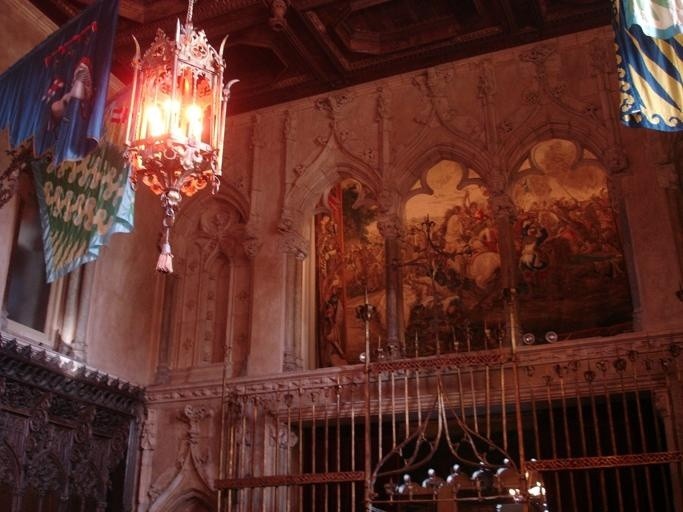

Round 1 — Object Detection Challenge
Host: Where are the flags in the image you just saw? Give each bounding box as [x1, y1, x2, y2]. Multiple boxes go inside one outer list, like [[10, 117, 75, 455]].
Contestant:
[[33, 84, 135, 284], [0, 0, 117, 167], [610, 1, 683, 132]]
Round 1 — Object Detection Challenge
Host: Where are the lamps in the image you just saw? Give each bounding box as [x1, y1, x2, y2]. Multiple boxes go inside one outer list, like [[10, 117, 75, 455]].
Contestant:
[[123, 0, 239, 275], [523, 331, 559, 344]]
